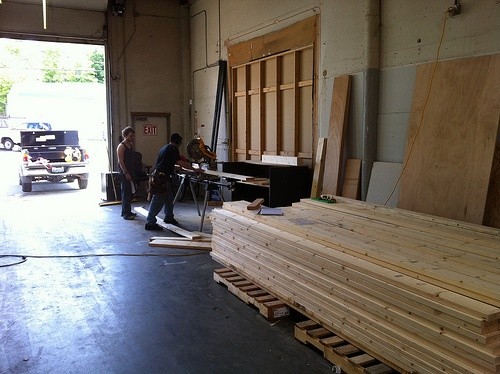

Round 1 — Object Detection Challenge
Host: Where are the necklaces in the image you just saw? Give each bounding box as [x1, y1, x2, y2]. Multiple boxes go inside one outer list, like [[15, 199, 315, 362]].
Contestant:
[[123, 139, 130, 146]]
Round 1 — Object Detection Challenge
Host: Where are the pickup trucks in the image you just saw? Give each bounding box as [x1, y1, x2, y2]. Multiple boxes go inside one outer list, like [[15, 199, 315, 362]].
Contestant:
[[19, 130, 89, 192]]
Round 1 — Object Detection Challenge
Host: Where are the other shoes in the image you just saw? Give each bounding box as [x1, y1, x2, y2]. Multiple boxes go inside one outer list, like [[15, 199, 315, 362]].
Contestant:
[[124, 213, 135, 220], [145, 224, 163, 231], [164, 216, 179, 226]]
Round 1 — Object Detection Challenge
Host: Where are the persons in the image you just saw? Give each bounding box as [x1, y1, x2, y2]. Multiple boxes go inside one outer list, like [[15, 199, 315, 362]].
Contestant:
[[145, 133, 205, 230], [117, 126, 142, 220]]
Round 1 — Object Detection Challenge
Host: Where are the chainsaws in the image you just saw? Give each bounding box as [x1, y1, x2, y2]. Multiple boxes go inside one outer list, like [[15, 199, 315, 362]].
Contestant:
[[187, 137, 218, 168]]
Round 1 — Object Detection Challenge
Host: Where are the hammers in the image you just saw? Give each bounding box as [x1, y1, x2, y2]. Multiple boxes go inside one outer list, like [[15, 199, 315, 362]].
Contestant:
[[146, 172, 153, 201]]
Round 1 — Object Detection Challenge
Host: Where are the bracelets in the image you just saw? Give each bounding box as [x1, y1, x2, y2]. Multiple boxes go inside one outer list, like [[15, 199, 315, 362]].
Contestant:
[[124, 169, 129, 176]]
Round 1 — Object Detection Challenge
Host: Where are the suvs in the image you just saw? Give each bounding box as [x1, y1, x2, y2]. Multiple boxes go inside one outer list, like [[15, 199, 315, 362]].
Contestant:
[[0, 120, 55, 150]]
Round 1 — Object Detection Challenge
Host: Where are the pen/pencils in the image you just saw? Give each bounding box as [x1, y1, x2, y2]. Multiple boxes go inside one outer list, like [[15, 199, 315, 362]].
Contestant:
[[257, 210, 261, 214]]
